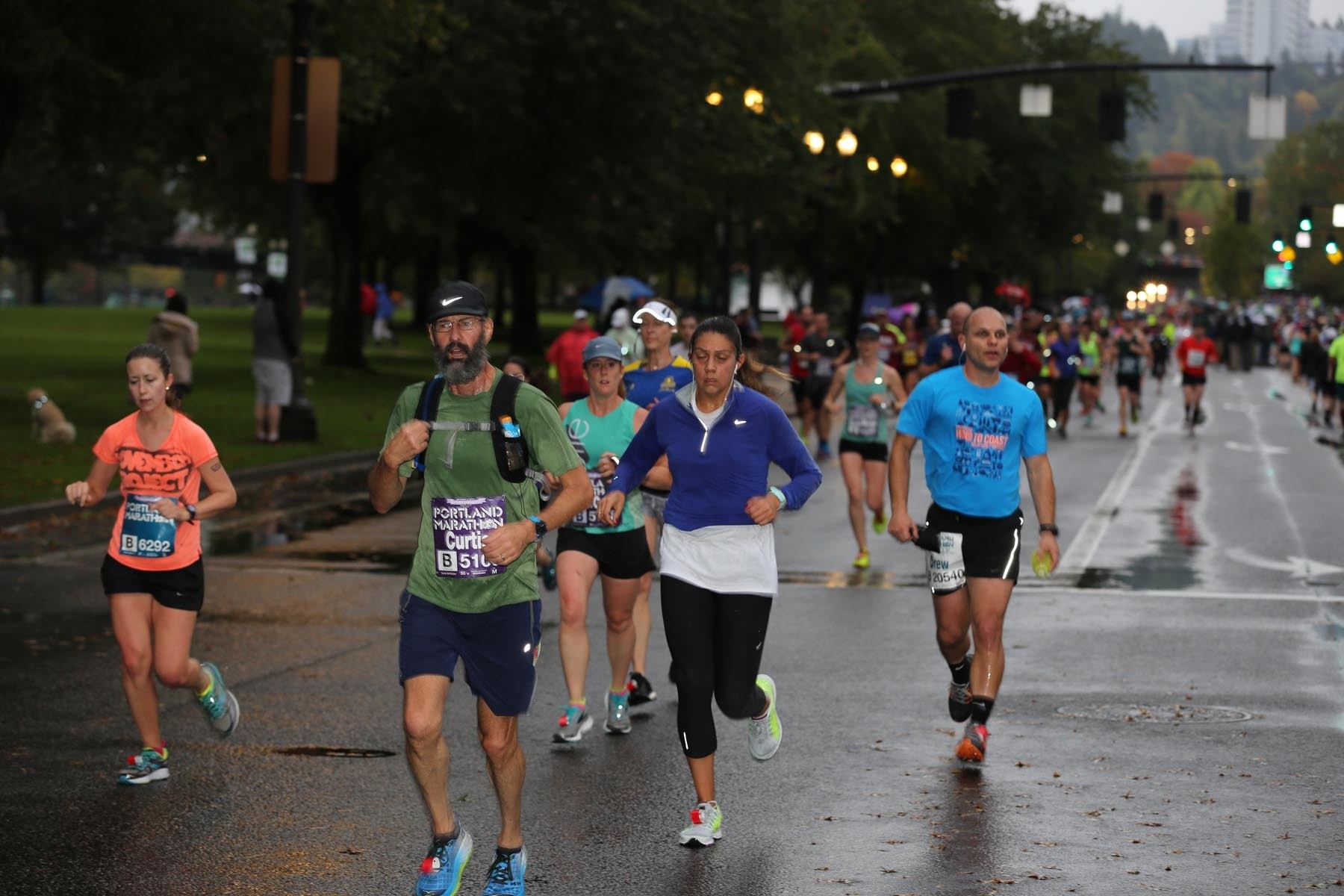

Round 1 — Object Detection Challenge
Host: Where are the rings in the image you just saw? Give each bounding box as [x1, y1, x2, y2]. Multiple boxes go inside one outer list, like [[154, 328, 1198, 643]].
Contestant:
[[500, 554, 505, 561], [598, 512, 601, 514]]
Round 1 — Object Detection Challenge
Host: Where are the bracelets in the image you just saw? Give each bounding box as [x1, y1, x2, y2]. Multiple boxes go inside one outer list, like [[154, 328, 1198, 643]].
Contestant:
[[891, 401, 896, 408], [768, 486, 785, 512]]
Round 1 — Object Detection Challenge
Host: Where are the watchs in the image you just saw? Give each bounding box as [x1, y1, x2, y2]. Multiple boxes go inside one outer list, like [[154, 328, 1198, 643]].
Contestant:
[[183, 504, 196, 525], [529, 515, 548, 539], [1040, 522, 1058, 538]]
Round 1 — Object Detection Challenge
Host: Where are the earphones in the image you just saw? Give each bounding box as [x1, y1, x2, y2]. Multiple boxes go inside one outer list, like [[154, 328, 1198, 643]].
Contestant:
[[962, 343, 966, 351], [734, 363, 738, 374]]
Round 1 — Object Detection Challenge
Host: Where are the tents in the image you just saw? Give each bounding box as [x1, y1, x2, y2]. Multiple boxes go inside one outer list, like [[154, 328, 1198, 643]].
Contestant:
[[573, 278, 653, 331]]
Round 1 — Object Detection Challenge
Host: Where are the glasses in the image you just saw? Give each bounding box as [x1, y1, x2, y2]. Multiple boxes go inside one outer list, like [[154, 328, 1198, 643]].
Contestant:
[[430, 318, 482, 332], [508, 373, 524, 382]]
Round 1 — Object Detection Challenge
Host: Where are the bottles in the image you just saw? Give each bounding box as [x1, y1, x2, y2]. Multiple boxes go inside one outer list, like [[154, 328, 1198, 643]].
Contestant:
[[1031, 550, 1053, 578]]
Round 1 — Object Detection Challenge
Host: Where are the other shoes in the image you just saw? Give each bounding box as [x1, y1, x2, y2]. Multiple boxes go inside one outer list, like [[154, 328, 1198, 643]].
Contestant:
[[853, 548, 869, 568], [255, 437, 281, 445], [873, 503, 887, 534], [814, 448, 830, 465], [541, 551, 557, 591]]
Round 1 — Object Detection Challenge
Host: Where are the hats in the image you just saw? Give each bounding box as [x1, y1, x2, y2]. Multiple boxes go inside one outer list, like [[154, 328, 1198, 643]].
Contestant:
[[573, 309, 587, 319], [856, 322, 880, 340], [581, 336, 622, 367], [632, 301, 678, 327], [610, 307, 629, 327], [424, 279, 488, 324]]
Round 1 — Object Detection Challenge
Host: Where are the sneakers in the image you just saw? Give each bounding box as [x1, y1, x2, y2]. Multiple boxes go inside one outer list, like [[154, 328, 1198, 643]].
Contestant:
[[191, 661, 240, 740], [603, 688, 632, 734], [482, 842, 528, 896], [416, 817, 473, 896], [955, 722, 988, 764], [678, 800, 723, 847], [551, 697, 593, 743], [748, 673, 782, 760], [116, 739, 169, 785], [628, 672, 657, 706], [948, 652, 975, 723]]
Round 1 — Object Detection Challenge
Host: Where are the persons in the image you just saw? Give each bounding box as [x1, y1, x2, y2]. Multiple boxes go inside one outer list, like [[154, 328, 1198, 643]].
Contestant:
[[65, 343, 239, 787], [150, 290, 1344, 895]]
